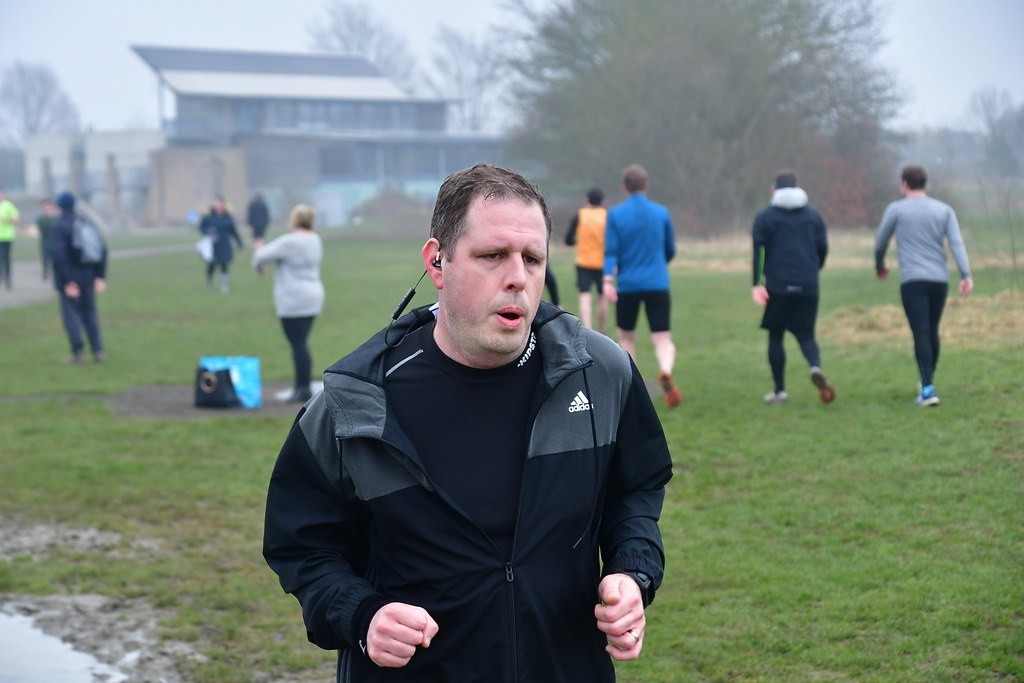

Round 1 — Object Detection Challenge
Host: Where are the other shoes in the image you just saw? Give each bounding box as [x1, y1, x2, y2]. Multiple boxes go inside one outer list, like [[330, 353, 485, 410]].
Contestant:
[[285, 386, 312, 404]]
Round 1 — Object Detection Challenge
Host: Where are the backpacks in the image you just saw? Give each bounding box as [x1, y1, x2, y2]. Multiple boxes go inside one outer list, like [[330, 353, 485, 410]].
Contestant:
[[62, 213, 107, 267]]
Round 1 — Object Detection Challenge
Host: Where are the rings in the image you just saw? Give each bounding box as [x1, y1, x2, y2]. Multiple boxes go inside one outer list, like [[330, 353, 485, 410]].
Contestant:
[[627, 629, 639, 643]]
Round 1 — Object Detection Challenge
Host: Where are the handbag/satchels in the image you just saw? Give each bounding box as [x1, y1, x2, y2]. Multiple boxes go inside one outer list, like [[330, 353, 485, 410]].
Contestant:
[[195, 233, 216, 264], [195, 356, 263, 409]]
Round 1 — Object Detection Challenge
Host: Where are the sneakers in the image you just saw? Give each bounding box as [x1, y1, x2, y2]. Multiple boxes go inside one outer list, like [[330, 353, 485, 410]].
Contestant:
[[916, 380, 940, 407], [658, 371, 681, 407], [809, 366, 836, 404], [763, 390, 788, 404]]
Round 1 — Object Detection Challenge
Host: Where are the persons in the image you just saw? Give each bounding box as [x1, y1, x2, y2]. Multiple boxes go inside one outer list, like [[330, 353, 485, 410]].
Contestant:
[[0, 189, 19, 293], [544, 265, 562, 309], [602, 163, 681, 408], [254, 205, 327, 405], [198, 192, 270, 292], [35, 189, 106, 365], [750, 170, 837, 403], [875, 165, 974, 405], [563, 188, 607, 334], [263, 166, 675, 683]]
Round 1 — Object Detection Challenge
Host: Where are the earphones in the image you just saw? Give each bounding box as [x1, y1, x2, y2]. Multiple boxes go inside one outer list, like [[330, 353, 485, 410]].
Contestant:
[[432, 254, 442, 267]]
